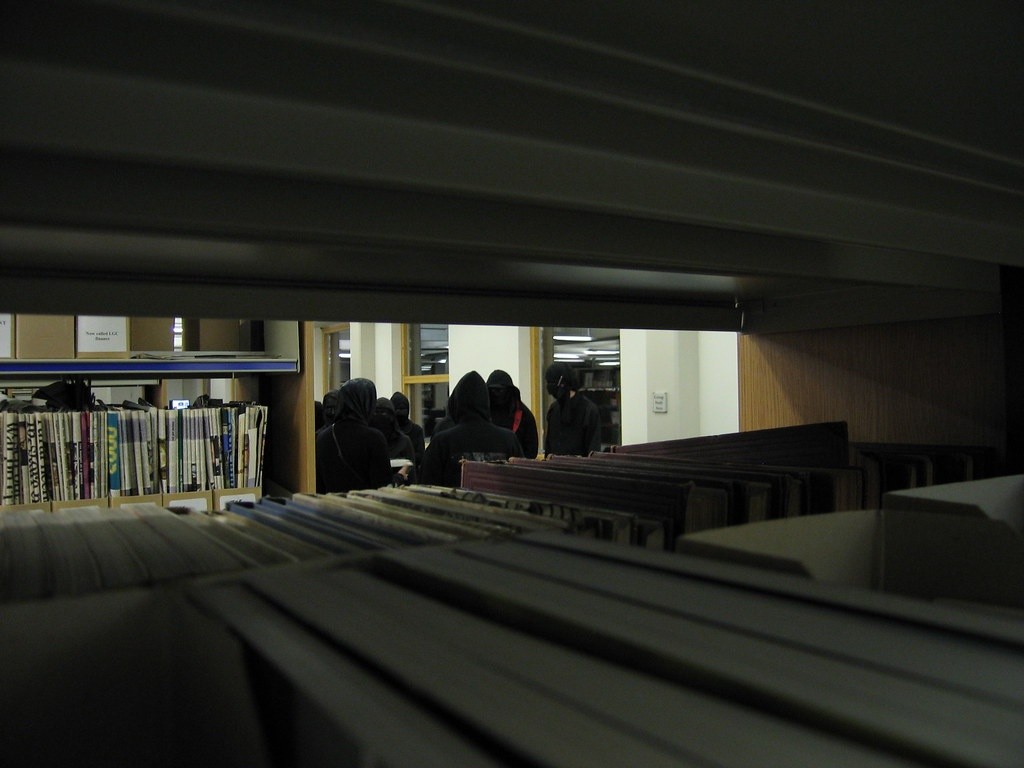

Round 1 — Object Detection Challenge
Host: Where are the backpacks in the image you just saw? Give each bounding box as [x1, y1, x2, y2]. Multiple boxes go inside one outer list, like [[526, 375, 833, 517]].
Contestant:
[[32, 378, 94, 412]]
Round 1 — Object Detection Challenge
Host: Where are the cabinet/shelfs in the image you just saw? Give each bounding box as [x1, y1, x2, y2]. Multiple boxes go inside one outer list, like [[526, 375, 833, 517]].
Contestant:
[[0, 312, 317, 521]]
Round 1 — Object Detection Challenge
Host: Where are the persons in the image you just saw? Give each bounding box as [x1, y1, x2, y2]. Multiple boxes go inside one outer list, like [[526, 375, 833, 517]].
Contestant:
[[315, 368, 540, 496], [539, 361, 603, 458]]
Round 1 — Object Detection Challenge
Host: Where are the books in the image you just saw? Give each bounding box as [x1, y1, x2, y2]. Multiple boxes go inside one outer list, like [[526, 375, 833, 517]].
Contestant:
[[0, 396, 1024, 768]]
